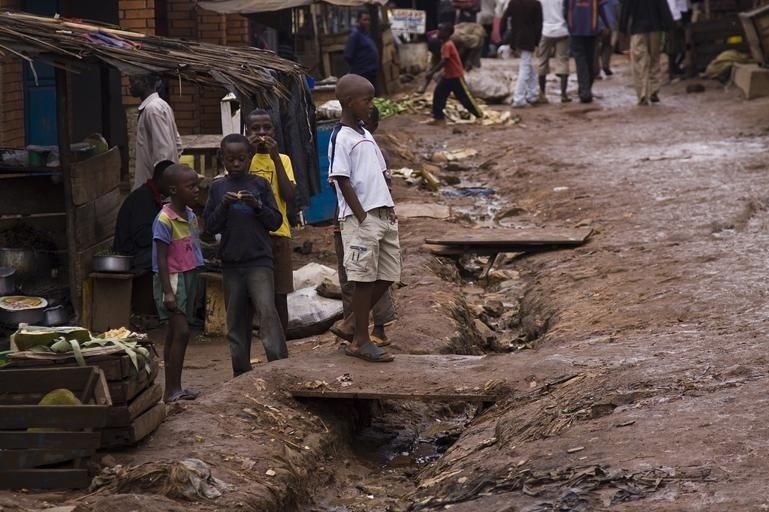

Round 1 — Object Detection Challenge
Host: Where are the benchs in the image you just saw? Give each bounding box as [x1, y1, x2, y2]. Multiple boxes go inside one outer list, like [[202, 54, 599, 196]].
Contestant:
[[86, 265, 149, 335]]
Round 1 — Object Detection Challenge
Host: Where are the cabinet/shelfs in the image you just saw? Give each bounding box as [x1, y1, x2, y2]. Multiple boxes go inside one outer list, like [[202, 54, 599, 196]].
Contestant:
[[737, 4, 769, 67]]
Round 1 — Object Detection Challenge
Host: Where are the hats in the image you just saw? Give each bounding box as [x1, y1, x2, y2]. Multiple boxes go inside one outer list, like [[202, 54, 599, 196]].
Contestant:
[[727, 36, 742, 44]]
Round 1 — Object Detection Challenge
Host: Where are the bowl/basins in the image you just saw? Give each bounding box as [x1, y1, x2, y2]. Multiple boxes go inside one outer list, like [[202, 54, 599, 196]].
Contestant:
[[0, 304, 65, 329], [91, 254, 134, 271]]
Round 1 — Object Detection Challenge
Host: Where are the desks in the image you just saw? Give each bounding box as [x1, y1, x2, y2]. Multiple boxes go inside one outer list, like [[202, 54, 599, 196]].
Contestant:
[[180, 135, 222, 177], [321, 43, 345, 78]]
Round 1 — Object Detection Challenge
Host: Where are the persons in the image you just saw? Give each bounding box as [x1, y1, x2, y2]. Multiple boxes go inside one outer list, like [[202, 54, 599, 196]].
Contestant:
[[111, 160, 175, 269], [360, 1, 498, 69], [620, 1, 692, 105], [151, 164, 205, 403], [332, 104, 399, 348], [328, 74, 402, 363], [425, 21, 483, 124], [128, 73, 184, 193], [203, 134, 288, 377], [498, 1, 619, 103], [245, 109, 297, 341], [343, 11, 379, 94]]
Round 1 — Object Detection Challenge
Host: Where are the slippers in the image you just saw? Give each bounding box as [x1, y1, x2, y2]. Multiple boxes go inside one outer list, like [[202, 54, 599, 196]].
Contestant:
[[641, 96, 659, 106], [163, 389, 197, 401], [369, 335, 391, 346], [603, 67, 613, 75], [329, 326, 354, 343], [513, 95, 592, 108], [344, 341, 395, 362]]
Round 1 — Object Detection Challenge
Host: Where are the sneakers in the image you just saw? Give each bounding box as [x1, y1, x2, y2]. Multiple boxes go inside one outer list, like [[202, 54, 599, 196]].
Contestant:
[[425, 118, 446, 126], [476, 113, 489, 125]]
